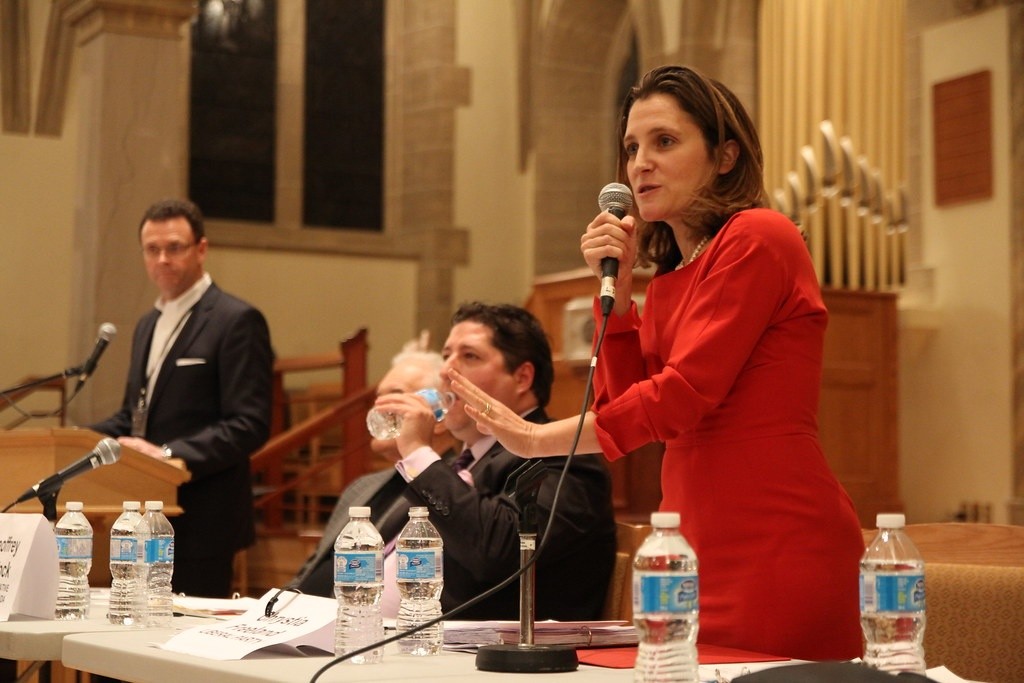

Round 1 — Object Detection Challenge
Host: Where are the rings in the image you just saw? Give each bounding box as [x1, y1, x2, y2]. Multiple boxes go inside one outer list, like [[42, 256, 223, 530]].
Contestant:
[[483, 402, 492, 416]]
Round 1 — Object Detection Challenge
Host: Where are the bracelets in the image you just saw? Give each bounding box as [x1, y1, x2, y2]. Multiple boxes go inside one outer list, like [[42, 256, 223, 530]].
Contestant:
[[161, 442, 174, 458]]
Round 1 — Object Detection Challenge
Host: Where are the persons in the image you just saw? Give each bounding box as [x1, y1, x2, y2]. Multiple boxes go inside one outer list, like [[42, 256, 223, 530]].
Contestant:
[[284, 346, 456, 598], [81, 197, 276, 599], [446, 66, 867, 663], [372, 302, 616, 621]]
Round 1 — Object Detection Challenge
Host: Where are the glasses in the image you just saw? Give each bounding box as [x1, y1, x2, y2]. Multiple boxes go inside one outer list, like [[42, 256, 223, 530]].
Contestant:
[[139, 244, 194, 257]]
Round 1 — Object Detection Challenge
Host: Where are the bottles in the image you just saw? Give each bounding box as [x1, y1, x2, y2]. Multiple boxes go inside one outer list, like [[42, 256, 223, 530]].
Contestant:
[[136, 501, 175, 627], [395, 507, 443, 654], [633, 512, 699, 683], [109, 501, 143, 625], [54, 501, 93, 622], [860, 514, 925, 675], [367, 387, 457, 440], [334, 507, 384, 664]]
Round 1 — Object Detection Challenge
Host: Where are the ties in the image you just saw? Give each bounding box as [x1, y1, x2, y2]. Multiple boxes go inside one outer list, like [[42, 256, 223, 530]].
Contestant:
[[382, 448, 475, 558]]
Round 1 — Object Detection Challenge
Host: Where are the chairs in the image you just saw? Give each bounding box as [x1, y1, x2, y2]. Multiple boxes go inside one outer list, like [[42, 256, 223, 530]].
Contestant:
[[598, 517, 1024, 682]]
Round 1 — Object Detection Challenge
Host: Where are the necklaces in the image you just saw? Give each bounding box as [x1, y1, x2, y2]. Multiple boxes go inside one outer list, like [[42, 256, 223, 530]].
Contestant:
[[675, 232, 710, 272]]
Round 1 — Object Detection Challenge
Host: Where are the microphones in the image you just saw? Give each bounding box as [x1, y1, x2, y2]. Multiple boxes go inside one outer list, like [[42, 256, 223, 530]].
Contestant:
[[598, 183, 633, 317], [16, 438, 123, 503], [75, 321, 115, 391]]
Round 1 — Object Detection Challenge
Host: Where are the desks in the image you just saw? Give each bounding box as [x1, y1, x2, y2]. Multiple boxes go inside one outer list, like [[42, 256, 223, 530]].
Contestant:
[[0, 584, 981, 683]]
